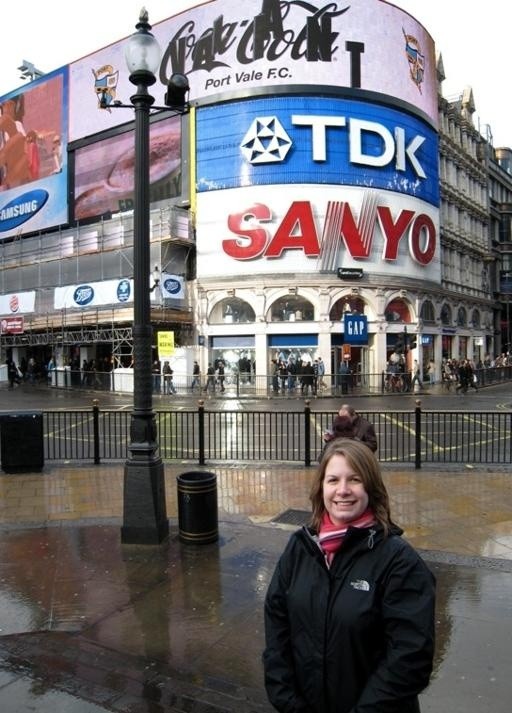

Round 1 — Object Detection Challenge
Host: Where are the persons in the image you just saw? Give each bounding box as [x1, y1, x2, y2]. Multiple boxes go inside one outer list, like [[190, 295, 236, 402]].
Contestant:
[[390, 351, 405, 373], [162, 361, 177, 395], [261, 435, 437, 712], [8, 356, 56, 389], [384, 359, 424, 394], [270, 356, 328, 395], [317, 416, 361, 465], [191, 361, 200, 392], [323, 403, 378, 454], [339, 360, 353, 394], [64, 356, 109, 388], [152, 360, 162, 395], [441, 350, 512, 396], [202, 362, 216, 392], [214, 356, 221, 369], [217, 362, 226, 391], [426, 359, 436, 385]]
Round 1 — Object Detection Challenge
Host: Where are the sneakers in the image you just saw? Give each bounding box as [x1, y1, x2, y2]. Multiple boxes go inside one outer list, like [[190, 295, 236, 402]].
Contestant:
[[167, 387, 225, 395]]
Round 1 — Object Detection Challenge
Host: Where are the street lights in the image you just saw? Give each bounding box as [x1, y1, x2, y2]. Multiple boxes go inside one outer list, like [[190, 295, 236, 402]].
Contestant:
[[100, 6, 191, 545]]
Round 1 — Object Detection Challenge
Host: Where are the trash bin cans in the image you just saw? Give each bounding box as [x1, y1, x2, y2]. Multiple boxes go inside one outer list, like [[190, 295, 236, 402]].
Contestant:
[[177, 471, 218, 545]]
